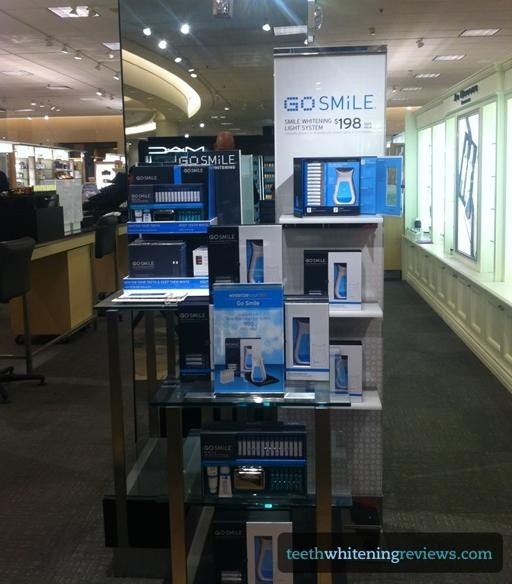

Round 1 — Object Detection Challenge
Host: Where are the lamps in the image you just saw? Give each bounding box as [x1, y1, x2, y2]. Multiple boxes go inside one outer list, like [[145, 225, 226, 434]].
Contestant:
[[27, 1, 122, 123], [144, 21, 271, 139]]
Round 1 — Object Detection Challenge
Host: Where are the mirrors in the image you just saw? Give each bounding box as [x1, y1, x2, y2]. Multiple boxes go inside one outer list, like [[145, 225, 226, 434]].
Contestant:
[[115, 0, 309, 166]]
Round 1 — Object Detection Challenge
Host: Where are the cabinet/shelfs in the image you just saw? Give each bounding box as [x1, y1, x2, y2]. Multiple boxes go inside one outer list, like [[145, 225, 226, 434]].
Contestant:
[[92, 45, 387, 583], [8, 144, 87, 187]]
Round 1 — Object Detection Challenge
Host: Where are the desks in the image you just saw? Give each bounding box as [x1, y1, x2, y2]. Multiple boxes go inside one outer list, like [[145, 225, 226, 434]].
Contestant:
[[8, 225, 127, 345]]
